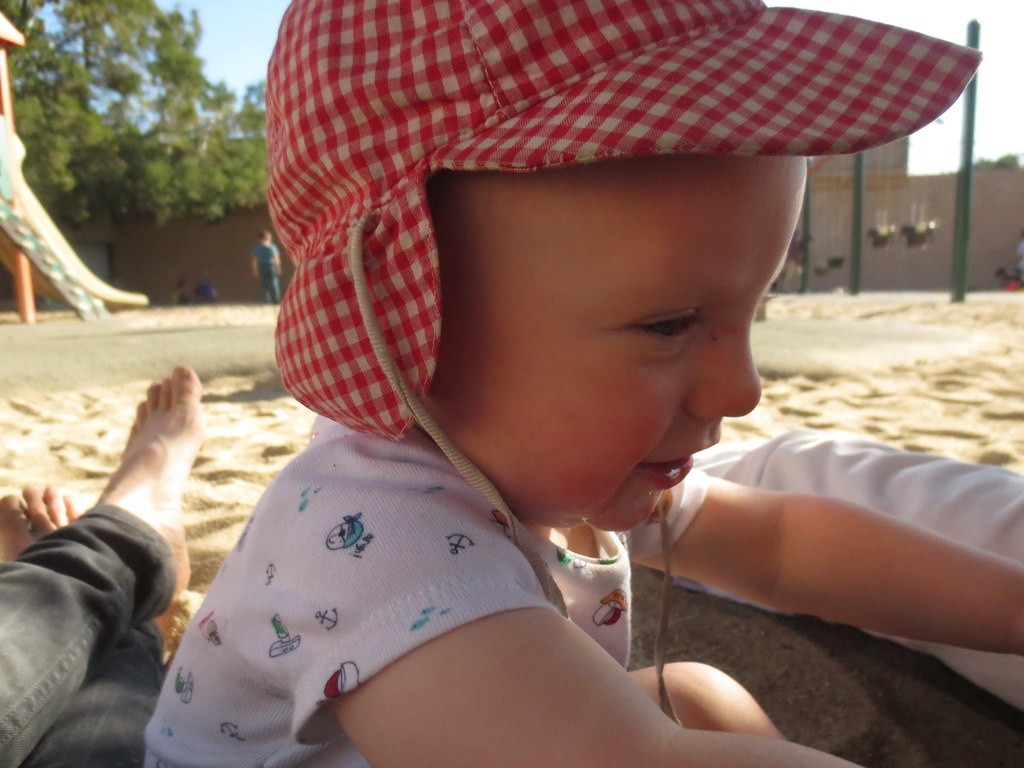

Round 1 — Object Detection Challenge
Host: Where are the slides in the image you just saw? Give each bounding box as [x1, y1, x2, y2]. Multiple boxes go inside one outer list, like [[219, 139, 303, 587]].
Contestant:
[[1, 115, 151, 311]]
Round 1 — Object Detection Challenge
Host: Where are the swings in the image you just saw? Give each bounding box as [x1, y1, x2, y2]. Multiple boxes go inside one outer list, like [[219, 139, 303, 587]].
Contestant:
[[813, 125, 935, 277]]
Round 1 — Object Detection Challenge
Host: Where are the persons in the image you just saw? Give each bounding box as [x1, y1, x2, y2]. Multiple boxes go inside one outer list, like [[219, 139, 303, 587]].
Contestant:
[[0, 365, 203, 768], [250, 231, 282, 304], [143, 1, 1024, 768]]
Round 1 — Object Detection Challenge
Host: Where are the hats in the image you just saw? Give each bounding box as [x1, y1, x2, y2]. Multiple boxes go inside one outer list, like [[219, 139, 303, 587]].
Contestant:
[[259, 0, 981, 442]]
